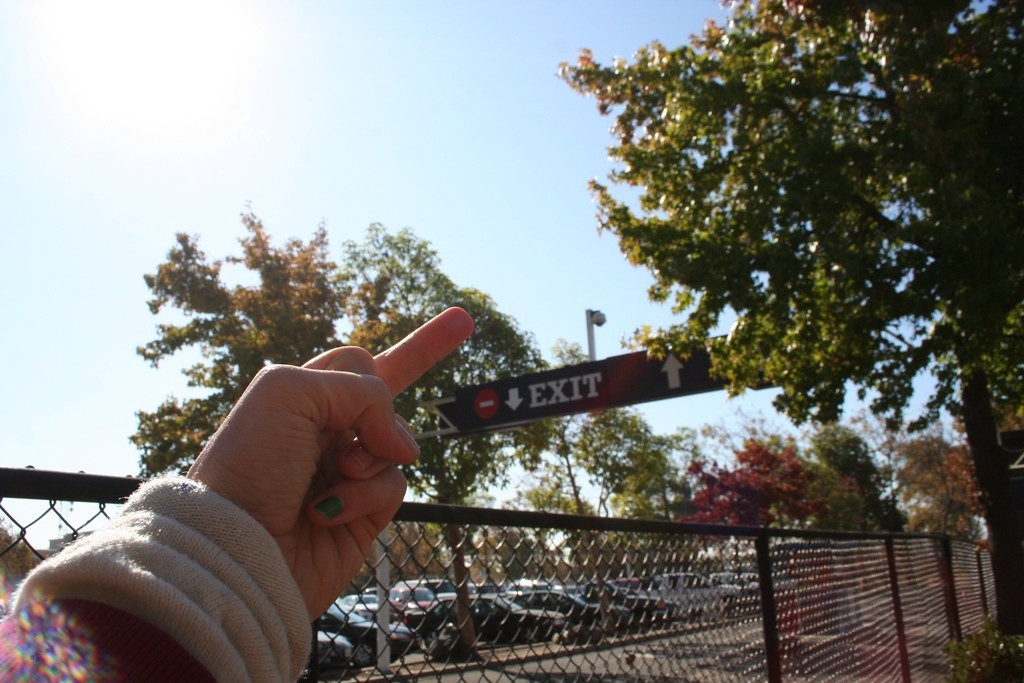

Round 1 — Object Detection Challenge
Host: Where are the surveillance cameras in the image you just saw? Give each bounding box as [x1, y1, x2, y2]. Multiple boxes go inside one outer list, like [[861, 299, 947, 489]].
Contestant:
[[593, 312, 606, 326]]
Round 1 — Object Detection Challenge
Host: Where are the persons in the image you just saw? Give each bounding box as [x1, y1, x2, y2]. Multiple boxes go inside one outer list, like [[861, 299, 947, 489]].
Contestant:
[[0, 307, 475, 683]]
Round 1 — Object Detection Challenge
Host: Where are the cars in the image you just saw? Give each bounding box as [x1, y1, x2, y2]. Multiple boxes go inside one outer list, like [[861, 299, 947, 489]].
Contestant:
[[309, 559, 798, 679]]
